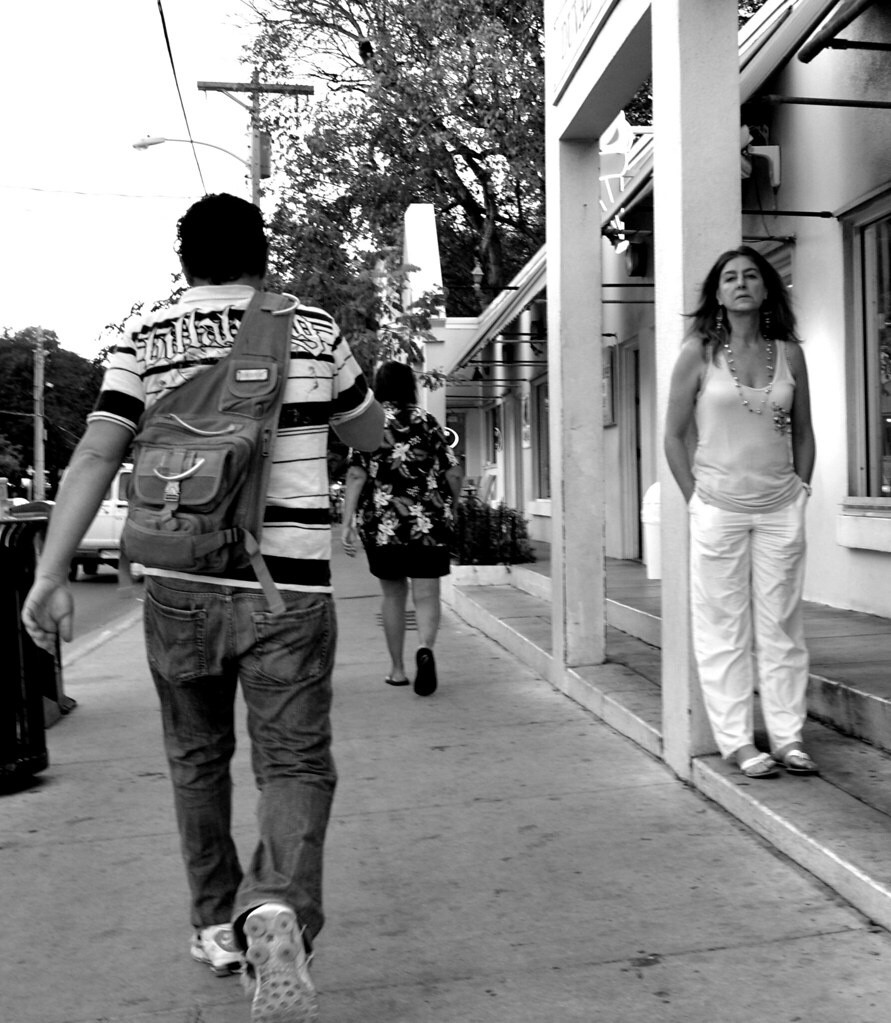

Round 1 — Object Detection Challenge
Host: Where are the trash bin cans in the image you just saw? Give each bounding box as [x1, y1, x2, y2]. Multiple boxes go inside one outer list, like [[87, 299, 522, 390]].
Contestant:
[[0, 516, 51, 786]]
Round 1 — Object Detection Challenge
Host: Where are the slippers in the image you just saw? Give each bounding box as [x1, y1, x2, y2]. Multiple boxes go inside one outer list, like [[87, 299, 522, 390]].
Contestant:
[[384, 672, 410, 686], [414, 648, 438, 697], [783, 747, 818, 776], [740, 753, 781, 779]]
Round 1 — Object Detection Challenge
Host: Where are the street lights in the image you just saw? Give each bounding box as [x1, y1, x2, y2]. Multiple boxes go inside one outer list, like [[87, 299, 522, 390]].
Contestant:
[[133, 137, 261, 220]]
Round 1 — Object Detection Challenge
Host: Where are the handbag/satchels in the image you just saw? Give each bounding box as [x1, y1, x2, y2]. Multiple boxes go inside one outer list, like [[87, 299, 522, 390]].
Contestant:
[[116, 289, 300, 578]]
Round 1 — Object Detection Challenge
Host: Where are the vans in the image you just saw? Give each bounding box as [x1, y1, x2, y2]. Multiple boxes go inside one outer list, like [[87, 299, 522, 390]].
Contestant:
[[68, 462, 134, 582]]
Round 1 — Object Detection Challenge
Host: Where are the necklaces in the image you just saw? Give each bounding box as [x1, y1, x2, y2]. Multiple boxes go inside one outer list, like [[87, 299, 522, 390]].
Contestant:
[[724, 334, 774, 414]]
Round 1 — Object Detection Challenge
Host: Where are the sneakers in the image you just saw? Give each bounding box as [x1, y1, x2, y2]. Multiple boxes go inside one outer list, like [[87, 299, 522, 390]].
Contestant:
[[190, 922, 248, 976], [244, 902, 316, 1023]]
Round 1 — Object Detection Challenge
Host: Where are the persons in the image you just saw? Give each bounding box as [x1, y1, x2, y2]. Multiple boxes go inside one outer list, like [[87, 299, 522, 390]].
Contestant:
[[20, 194, 385, 1023], [342, 362, 464, 697], [664, 249, 819, 776]]
